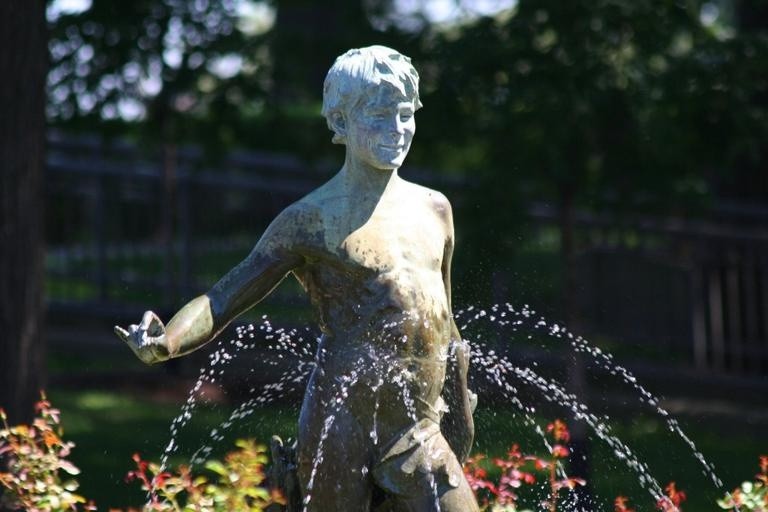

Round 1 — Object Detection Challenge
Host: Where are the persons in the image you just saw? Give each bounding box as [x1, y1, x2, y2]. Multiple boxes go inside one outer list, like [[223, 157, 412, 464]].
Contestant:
[[112, 44, 483, 512]]
